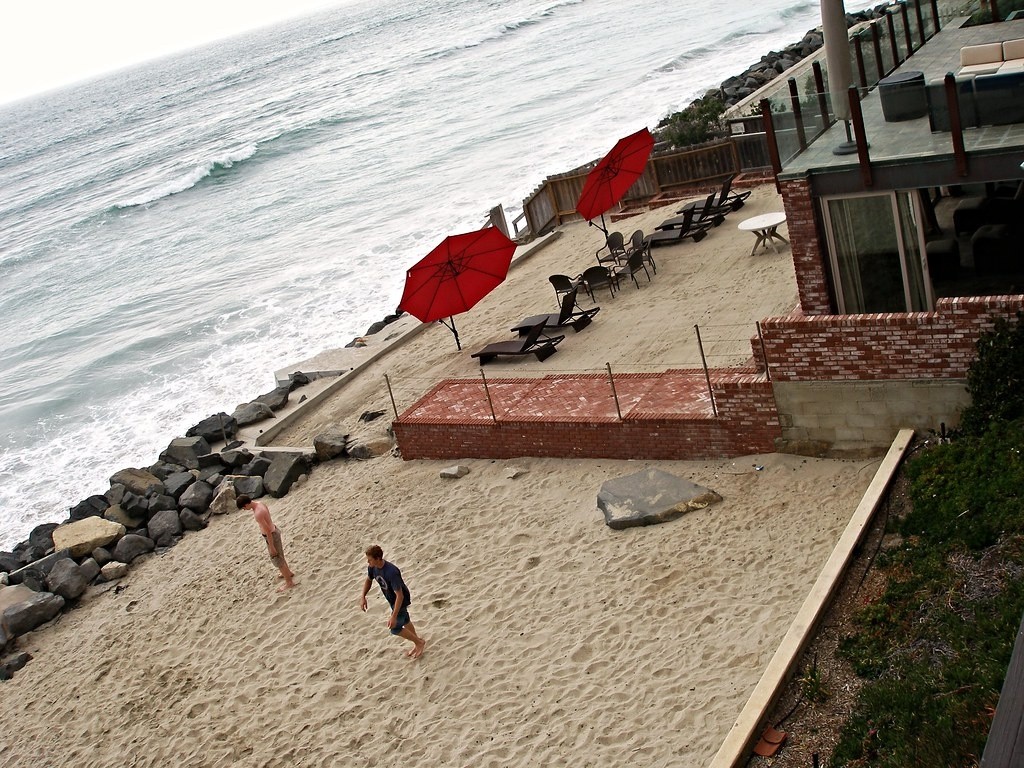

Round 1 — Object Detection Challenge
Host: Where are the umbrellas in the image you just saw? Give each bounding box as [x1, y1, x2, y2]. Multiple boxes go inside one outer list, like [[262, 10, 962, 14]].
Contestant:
[[396, 224, 518, 349], [576, 127, 658, 242]]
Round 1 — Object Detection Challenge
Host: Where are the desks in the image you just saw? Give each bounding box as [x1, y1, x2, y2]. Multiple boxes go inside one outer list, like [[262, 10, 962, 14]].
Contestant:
[[878, 71, 927, 122], [738, 212, 787, 256]]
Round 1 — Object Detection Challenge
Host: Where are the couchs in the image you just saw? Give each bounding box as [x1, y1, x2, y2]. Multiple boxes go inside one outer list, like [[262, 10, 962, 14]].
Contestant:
[[925, 38, 1024, 134], [989, 181, 1024, 208]]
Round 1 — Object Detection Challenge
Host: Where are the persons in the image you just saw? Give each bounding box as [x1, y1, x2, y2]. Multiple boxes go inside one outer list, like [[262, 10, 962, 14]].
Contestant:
[[234, 494, 294, 587], [359, 545, 427, 659]]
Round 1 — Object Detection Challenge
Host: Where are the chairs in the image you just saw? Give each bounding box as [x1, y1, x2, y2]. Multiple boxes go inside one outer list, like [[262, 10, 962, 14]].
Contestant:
[[583, 266, 616, 303], [510, 287, 600, 338], [643, 203, 707, 242], [676, 174, 751, 211], [596, 232, 626, 266], [613, 249, 650, 290], [654, 191, 725, 228], [549, 273, 590, 308], [629, 235, 656, 281], [615, 230, 643, 266], [471, 316, 565, 366]]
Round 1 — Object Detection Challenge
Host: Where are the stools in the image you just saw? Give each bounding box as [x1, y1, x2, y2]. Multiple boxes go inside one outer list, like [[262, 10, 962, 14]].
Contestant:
[[970, 224, 1008, 274], [953, 196, 987, 239], [926, 239, 960, 268]]
